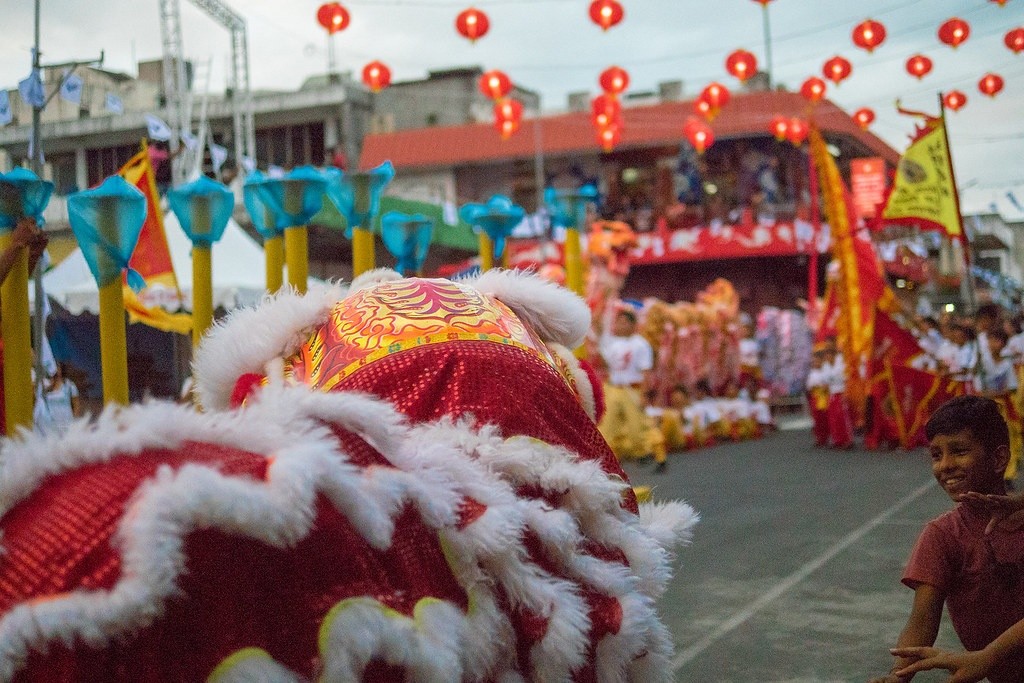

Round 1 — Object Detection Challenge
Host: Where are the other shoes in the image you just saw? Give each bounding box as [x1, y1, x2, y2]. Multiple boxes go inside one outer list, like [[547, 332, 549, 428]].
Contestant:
[[658, 463, 667, 473]]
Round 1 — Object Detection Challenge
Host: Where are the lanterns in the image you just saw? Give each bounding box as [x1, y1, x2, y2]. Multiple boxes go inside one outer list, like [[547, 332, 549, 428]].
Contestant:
[[317, 0, 1024, 152]]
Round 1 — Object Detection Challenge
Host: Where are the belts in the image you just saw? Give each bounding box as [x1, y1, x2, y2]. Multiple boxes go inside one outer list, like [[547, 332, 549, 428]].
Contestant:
[[616, 384, 642, 388]]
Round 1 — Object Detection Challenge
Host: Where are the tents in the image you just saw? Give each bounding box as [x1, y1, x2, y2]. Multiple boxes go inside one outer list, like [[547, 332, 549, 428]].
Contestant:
[[35, 185, 326, 405]]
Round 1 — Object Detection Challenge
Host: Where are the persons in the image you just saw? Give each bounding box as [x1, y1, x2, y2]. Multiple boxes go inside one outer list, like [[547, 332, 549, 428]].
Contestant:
[[580, 255, 1024, 493], [869, 393, 1024, 683], [888, 615, 1024, 683]]
